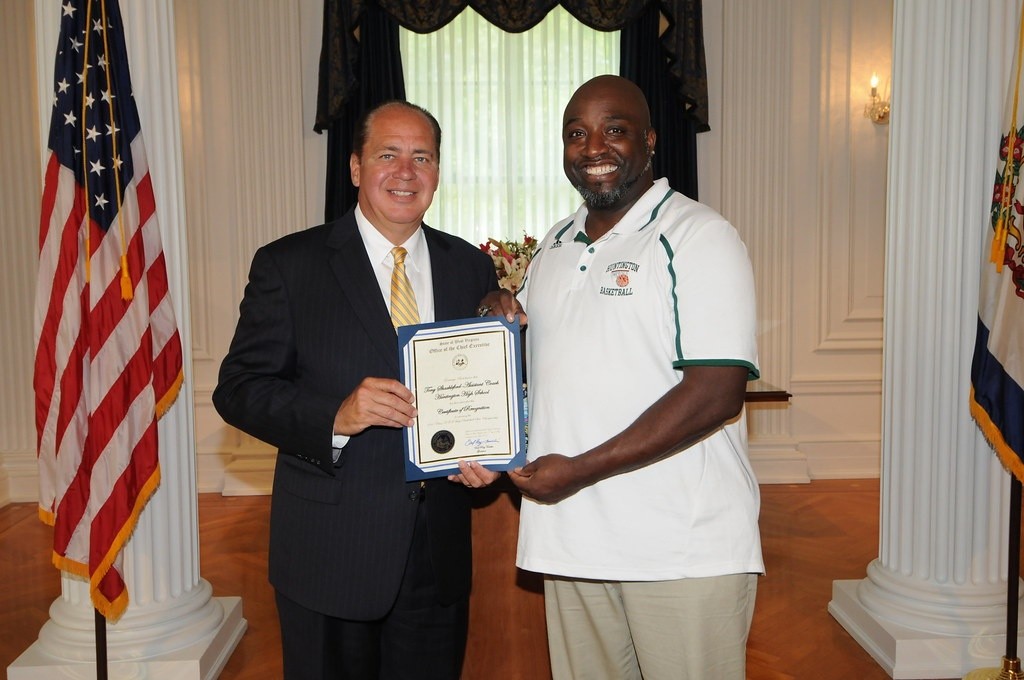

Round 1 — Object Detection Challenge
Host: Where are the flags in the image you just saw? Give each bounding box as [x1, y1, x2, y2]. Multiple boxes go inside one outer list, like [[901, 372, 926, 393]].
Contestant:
[[33, 1, 184, 625], [968, 0, 1024, 484]]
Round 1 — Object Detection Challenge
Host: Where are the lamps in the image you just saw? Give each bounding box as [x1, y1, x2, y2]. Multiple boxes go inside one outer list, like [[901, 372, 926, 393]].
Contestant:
[[864, 70, 891, 125]]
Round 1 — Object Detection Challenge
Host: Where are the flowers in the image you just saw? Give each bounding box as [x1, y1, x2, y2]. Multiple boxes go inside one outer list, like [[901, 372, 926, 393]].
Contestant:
[[479, 234, 538, 295]]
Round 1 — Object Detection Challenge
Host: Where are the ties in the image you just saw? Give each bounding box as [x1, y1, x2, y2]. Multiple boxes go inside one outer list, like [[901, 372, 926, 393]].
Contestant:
[[390, 246, 420, 328]]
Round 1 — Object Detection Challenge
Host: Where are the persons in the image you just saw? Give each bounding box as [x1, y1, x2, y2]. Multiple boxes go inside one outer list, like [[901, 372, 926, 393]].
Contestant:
[[477, 75, 767, 680], [212, 100, 501, 680]]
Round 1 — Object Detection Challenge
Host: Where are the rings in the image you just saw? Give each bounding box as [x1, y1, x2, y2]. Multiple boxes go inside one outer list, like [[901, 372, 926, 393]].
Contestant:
[[477, 303, 491, 316]]
